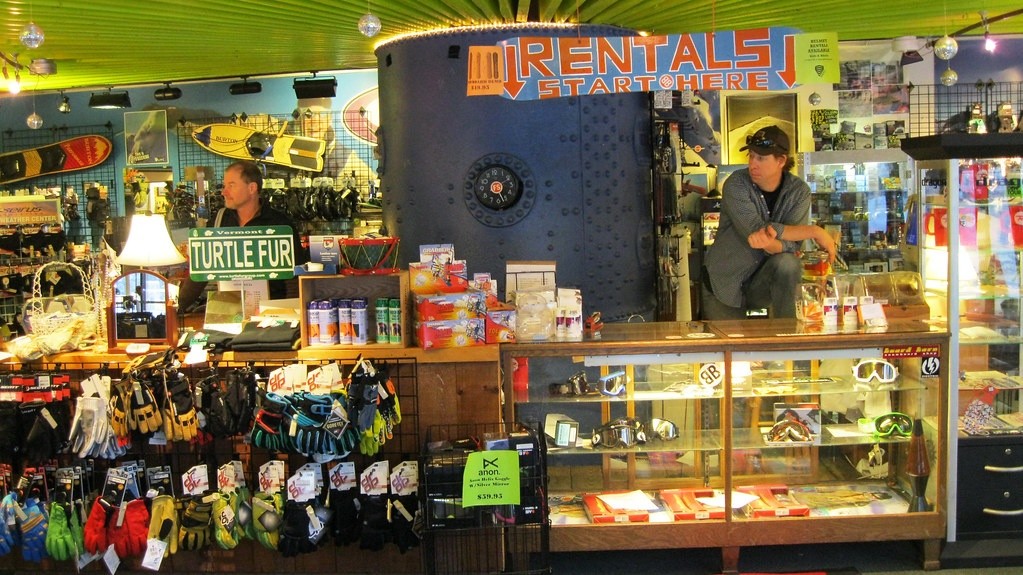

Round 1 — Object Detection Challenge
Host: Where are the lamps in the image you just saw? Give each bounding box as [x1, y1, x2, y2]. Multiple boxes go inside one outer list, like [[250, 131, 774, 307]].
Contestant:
[[292, 71, 338, 99], [359, 0, 381, 38], [934, 1, 959, 59], [89, 88, 132, 110], [984, 17, 994, 50], [154, 82, 182, 100], [230, 76, 262, 95], [116, 213, 188, 272], [809, 91, 820, 105], [20, 1, 45, 48], [940, 59, 958, 87], [58, 91, 71, 114], [2, 65, 20, 94], [27, 93, 44, 130]]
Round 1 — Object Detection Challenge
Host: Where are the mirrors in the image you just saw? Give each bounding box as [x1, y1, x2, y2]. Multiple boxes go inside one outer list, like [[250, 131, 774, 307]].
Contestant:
[[107, 269, 168, 353]]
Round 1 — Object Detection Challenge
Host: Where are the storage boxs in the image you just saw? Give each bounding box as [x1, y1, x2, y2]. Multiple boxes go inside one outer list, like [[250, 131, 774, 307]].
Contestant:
[[736, 486, 808, 516], [660, 489, 725, 521], [408, 245, 583, 349], [582, 491, 650, 524], [299, 271, 411, 350], [545, 414, 580, 447], [774, 402, 822, 434]]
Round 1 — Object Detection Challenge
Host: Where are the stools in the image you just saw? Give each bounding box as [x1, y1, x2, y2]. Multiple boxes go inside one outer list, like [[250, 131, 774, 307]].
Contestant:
[[741, 358, 819, 474]]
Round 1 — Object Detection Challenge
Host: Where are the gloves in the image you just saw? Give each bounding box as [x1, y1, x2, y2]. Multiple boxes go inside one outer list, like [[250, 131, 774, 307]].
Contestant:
[[0, 366, 420, 560]]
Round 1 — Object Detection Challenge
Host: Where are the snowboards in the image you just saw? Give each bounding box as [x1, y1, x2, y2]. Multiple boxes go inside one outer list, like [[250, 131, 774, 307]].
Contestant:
[[191, 124, 327, 172], [0, 135, 112, 185]]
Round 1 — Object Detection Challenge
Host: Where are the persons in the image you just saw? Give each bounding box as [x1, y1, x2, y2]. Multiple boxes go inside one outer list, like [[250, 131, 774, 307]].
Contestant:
[[205, 161, 305, 300], [701, 126, 835, 320]]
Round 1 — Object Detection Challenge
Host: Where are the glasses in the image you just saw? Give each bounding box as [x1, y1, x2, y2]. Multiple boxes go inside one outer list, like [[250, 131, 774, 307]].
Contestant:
[[851, 359, 899, 383], [858, 413, 915, 436], [549, 371, 591, 395], [592, 416, 679, 448], [598, 371, 627, 396], [746, 135, 788, 150], [771, 421, 814, 443]]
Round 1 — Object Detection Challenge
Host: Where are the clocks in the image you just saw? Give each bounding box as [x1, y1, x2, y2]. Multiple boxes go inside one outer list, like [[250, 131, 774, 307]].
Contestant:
[[475, 168, 516, 207]]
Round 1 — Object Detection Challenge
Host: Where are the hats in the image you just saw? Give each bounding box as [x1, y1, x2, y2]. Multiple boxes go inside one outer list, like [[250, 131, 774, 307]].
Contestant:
[[739, 125, 789, 152]]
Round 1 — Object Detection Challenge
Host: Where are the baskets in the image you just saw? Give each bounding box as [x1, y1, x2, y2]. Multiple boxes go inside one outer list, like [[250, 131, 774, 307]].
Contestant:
[[29, 261, 97, 336]]
